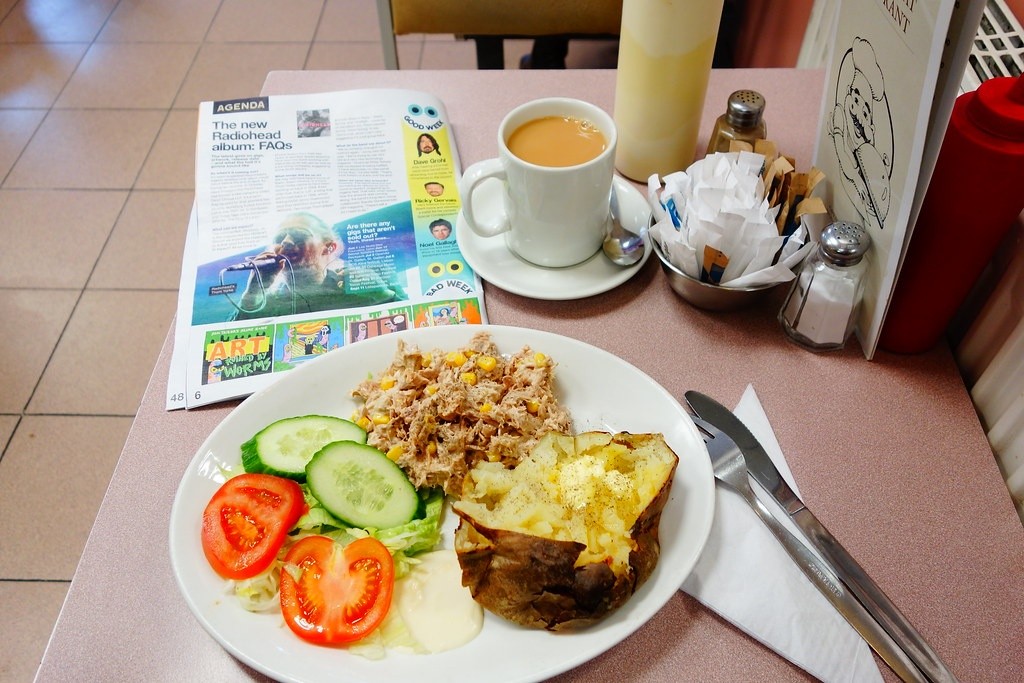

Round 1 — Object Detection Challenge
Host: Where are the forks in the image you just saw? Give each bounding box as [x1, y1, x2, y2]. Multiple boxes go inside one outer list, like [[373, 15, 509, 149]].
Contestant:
[[686, 413, 929, 683]]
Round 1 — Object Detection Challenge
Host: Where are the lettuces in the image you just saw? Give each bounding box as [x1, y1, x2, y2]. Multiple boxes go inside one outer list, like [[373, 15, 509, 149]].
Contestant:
[[215, 464, 443, 655]]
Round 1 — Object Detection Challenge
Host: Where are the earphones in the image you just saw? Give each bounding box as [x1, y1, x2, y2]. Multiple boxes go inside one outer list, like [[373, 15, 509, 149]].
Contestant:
[[326, 245, 333, 252]]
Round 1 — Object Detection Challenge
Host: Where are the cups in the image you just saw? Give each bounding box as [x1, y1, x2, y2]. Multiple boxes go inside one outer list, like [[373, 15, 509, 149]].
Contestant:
[[459, 97, 618, 268]]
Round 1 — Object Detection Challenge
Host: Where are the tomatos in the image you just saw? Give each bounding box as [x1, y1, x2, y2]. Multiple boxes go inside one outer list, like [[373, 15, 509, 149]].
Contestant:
[[200, 473, 307, 580], [278, 536, 394, 645]]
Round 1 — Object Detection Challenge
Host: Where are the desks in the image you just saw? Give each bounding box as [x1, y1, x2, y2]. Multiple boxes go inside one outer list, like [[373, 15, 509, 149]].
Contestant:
[[33, 67, 1023, 683]]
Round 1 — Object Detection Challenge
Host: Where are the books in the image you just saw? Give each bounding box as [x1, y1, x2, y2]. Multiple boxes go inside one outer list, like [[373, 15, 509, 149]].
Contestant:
[[165, 89, 491, 411]]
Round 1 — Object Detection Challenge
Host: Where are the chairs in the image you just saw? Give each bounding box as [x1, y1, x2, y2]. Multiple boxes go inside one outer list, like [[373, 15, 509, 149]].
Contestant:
[[376, 0, 624, 69]]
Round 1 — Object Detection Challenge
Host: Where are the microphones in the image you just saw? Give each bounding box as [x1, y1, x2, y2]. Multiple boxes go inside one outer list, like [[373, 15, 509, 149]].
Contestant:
[[226, 255, 285, 271]]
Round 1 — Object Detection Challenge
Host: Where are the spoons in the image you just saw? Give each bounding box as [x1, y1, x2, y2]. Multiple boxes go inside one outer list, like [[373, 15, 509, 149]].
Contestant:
[[602, 183, 645, 266]]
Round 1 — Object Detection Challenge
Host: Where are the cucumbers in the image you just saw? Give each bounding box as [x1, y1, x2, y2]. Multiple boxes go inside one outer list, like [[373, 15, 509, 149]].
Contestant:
[[305, 440, 427, 530], [240, 415, 369, 483]]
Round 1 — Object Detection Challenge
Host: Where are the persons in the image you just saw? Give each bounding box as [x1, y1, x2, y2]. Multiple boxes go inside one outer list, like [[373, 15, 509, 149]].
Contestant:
[[428, 218, 451, 241], [417, 133, 441, 158], [225, 212, 404, 321], [424, 181, 444, 197]]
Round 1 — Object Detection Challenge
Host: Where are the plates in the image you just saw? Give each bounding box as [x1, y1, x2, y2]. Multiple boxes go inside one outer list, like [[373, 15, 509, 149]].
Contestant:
[[454, 174, 654, 301], [167, 325, 715, 683]]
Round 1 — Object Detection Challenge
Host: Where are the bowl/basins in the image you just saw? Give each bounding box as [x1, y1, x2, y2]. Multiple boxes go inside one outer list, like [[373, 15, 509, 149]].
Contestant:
[[648, 207, 804, 310]]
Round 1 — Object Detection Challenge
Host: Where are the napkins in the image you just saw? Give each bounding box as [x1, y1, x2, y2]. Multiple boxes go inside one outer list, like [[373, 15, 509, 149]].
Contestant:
[[678, 384, 884, 683]]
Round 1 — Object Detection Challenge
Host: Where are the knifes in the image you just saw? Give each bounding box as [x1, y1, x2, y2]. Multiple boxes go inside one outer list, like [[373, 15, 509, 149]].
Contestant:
[[686, 389, 963, 683]]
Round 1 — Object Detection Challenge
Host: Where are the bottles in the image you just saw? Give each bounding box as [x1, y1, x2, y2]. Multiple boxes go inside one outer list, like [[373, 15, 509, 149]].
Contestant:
[[777, 221, 872, 351], [703, 89, 767, 158], [878, 68, 1024, 357]]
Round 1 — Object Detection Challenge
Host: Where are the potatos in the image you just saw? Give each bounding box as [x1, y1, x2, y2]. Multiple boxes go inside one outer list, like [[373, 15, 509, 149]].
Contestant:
[[450, 430, 679, 633]]
